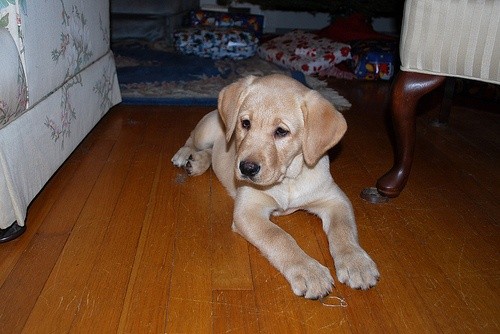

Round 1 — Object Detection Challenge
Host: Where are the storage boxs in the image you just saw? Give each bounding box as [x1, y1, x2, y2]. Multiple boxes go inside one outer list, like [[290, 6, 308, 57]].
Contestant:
[[258, 29, 352, 77], [176, 25, 257, 58]]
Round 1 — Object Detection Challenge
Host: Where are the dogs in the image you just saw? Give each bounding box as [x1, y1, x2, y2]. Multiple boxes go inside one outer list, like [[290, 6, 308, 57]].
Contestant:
[[171, 74, 381, 300]]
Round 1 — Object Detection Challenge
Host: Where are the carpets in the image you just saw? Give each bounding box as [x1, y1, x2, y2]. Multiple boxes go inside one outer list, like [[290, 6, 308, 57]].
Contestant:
[[111, 39, 358, 111]]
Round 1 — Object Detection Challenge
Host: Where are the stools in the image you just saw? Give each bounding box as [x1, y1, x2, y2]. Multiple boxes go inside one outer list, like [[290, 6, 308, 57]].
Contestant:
[[375, 0, 500, 198]]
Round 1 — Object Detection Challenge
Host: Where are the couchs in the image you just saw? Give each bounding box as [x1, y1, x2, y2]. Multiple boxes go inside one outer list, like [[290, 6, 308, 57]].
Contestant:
[[110, 0, 200, 39], [0, 0, 123, 243]]
